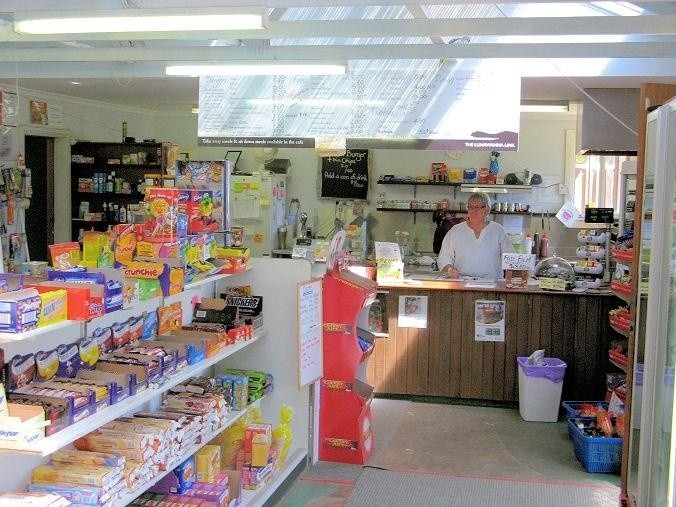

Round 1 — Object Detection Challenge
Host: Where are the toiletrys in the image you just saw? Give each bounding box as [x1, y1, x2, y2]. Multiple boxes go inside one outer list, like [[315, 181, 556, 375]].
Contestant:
[[91, 171, 116, 193], [102, 202, 126, 223]]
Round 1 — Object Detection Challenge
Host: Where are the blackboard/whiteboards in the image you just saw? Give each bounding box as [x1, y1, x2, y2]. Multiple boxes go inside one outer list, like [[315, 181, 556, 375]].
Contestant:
[[296, 275, 324, 391], [317, 148, 372, 203]]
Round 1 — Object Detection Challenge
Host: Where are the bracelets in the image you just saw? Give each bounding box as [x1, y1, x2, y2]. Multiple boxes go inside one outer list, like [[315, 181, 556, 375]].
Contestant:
[[447, 266, 454, 273]]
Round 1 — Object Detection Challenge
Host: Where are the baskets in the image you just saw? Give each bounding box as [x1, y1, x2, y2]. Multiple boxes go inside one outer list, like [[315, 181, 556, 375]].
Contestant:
[[561, 400, 628, 473]]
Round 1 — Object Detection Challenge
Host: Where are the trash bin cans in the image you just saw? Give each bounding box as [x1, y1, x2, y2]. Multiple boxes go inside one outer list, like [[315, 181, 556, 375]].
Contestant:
[[517, 357, 565, 422]]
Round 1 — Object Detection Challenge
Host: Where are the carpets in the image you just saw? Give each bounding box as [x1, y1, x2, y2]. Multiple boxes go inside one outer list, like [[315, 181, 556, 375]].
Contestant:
[[345, 466, 621, 507]]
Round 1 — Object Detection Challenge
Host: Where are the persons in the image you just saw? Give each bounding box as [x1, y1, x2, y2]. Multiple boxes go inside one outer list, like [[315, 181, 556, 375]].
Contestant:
[[436, 191, 516, 279]]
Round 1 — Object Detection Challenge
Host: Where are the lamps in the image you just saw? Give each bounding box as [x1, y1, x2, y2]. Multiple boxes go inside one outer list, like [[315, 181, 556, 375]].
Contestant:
[[521, 100, 569, 112], [13, 6, 270, 35], [164, 59, 349, 77]]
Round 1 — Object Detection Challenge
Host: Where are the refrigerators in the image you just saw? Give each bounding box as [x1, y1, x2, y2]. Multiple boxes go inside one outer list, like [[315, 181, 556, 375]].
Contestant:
[[228, 171, 287, 260]]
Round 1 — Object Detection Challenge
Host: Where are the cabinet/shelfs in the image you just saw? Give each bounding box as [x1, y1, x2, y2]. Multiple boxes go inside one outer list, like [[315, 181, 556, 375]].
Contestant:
[[318, 270, 378, 465], [70, 142, 166, 250], [0, 258, 311, 507], [604, 250, 633, 408], [377, 179, 534, 224]]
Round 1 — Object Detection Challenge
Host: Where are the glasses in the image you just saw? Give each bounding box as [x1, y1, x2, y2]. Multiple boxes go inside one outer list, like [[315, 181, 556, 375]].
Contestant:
[[466, 204, 486, 209]]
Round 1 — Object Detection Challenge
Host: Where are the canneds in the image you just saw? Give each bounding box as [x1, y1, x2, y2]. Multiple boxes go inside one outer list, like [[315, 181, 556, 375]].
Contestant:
[[523, 169, 531, 185], [491, 201, 519, 211], [454, 202, 467, 211]]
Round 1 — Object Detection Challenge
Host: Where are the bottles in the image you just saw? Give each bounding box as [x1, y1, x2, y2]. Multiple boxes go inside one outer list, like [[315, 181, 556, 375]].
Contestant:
[[525, 228, 550, 257], [92, 171, 116, 193], [101, 201, 127, 223]]
